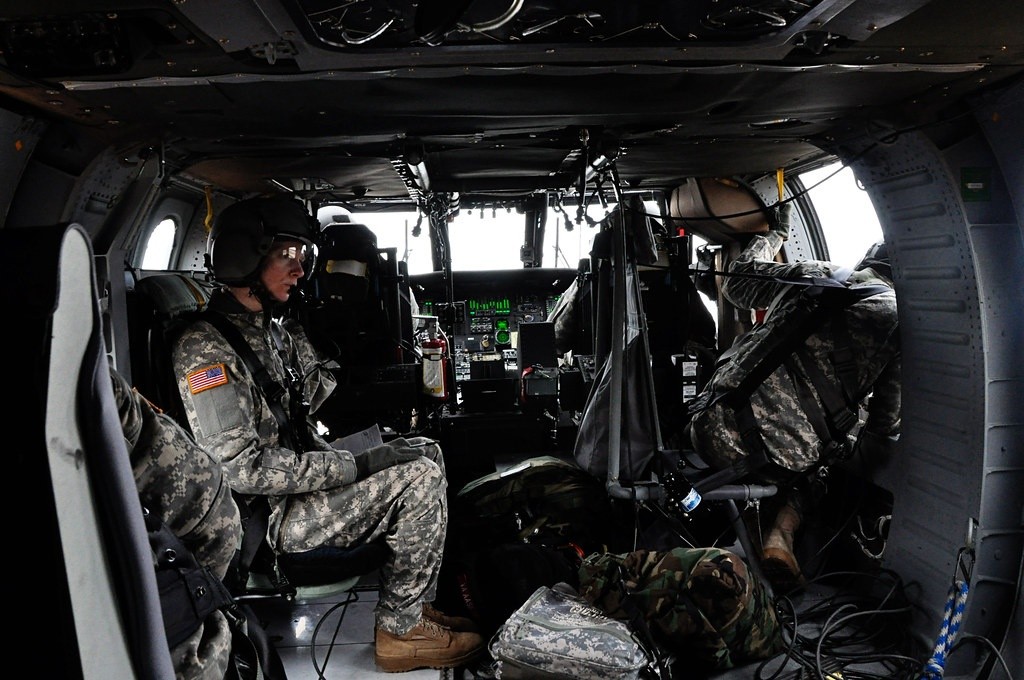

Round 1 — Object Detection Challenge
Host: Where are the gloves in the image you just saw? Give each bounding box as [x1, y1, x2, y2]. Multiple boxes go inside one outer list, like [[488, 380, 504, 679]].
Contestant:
[[352, 437, 426, 483], [763, 201, 790, 233]]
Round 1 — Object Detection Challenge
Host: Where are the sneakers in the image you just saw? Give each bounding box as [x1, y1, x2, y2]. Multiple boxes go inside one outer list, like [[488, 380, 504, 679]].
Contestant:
[[420, 603, 471, 631], [374, 615, 482, 672]]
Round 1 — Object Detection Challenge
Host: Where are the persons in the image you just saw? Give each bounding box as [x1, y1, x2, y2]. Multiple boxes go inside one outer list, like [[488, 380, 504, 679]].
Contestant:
[[158, 191, 480, 672], [688, 240, 902, 583], [108, 364, 243, 680]]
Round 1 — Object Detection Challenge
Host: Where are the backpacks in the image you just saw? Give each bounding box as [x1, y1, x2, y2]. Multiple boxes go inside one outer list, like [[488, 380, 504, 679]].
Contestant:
[[489, 581, 675, 680]]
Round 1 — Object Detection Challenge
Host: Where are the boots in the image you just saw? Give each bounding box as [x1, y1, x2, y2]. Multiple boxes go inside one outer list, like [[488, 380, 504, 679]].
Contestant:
[[761, 507, 809, 591]]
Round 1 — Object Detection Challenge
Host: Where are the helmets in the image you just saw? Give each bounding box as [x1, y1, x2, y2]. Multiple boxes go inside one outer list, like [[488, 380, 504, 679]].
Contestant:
[[212, 197, 318, 287], [855, 239, 890, 273]]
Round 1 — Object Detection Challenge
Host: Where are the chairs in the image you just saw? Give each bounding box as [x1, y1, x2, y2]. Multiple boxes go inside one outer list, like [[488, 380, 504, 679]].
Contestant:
[[574, 220, 689, 409], [307, 223, 403, 425], [0, 220, 270, 680], [133, 273, 384, 600]]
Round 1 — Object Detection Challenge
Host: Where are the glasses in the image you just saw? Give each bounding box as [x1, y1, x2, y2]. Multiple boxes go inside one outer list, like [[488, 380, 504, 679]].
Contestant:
[[270, 248, 307, 269]]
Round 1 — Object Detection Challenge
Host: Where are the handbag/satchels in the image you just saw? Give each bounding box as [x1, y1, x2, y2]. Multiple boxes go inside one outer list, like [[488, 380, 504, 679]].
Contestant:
[[578, 547, 785, 677]]
